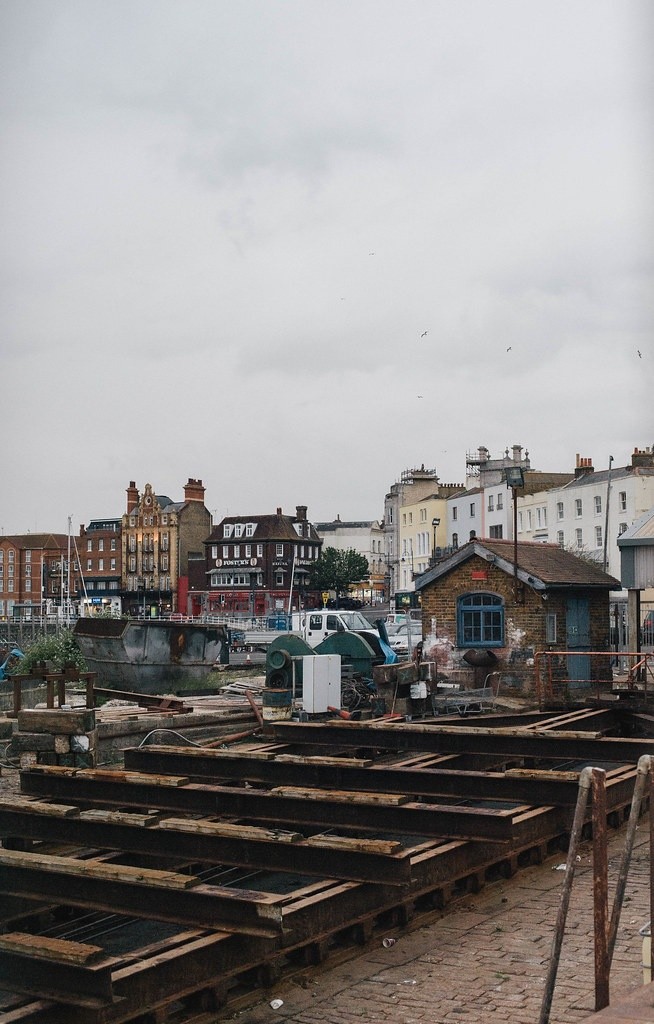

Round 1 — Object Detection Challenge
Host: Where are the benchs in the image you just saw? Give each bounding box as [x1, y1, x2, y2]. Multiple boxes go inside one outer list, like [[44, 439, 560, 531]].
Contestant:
[[311, 623, 335, 630]]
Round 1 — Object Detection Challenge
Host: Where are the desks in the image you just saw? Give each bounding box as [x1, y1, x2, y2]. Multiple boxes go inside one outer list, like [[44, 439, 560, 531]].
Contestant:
[[10, 672, 98, 718]]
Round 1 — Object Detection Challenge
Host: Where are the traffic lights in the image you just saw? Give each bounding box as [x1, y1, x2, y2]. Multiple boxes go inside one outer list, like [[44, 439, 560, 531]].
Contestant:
[[198, 597, 201, 606], [218, 594, 221, 604], [250, 593, 254, 604], [201, 596, 205, 605], [221, 594, 225, 603]]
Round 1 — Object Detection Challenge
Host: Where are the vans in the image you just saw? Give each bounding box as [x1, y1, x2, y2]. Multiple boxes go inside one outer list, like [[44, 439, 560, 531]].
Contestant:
[[382, 608, 423, 637]]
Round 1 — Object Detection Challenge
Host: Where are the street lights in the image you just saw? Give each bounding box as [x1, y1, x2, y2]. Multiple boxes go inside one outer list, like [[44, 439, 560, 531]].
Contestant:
[[502, 466, 525, 601], [602, 454, 614, 573], [149, 578, 155, 619]]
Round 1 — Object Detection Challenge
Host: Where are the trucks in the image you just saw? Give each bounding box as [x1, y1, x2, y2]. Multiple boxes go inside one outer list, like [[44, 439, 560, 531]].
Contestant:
[[243, 611, 379, 653]]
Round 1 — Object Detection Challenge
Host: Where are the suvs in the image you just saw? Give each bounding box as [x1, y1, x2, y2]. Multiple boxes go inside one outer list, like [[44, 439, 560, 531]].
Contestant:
[[332, 597, 363, 610], [643, 610, 654, 645]]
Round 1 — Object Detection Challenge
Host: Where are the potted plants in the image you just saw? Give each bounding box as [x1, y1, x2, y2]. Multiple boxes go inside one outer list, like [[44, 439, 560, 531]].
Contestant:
[[10, 633, 49, 676], [50, 645, 88, 674]]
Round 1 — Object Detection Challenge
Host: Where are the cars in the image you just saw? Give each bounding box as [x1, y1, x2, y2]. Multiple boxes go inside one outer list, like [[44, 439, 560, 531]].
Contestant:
[[387, 622, 422, 656]]
